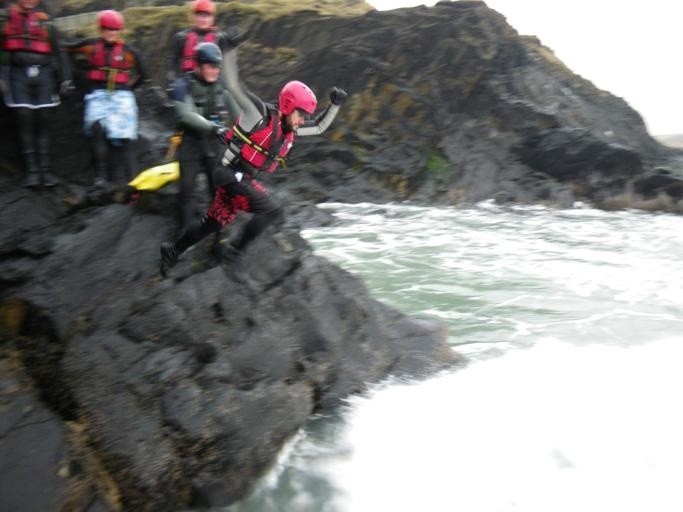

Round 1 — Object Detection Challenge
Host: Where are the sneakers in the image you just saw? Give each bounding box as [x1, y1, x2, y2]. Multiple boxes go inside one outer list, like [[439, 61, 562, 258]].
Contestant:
[[22, 174, 58, 187], [160, 241, 178, 278]]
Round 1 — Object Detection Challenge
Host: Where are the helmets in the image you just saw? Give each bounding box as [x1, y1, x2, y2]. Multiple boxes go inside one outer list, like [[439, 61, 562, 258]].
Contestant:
[[97, 9, 124, 29], [192, 0, 215, 14], [279, 80, 318, 115], [193, 42, 223, 67]]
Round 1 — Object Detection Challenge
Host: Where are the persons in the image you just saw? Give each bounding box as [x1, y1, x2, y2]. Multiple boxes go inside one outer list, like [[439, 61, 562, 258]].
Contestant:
[[0, 0, 74, 188], [78, 8, 147, 198], [159, 1, 348, 279]]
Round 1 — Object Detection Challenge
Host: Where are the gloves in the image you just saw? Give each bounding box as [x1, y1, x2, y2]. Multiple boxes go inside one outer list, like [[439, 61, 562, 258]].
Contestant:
[[330, 86, 347, 105], [212, 125, 230, 145], [218, 31, 239, 51]]
